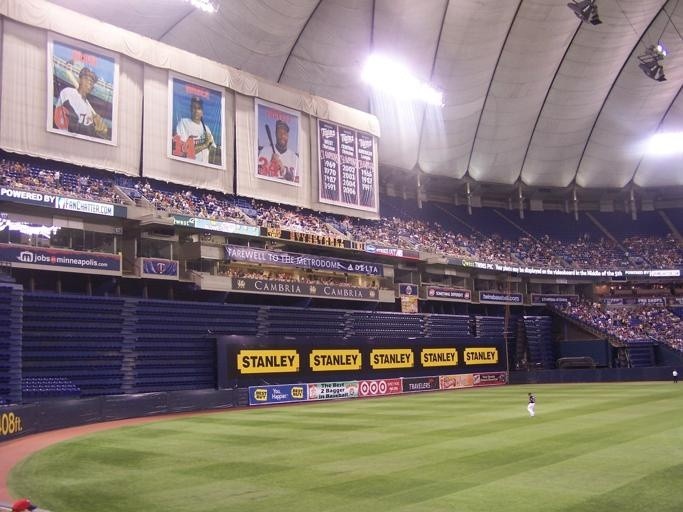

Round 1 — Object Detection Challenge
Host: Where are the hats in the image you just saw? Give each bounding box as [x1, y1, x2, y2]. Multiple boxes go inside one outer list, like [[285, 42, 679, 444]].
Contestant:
[[276, 120, 289, 133], [79, 67, 98, 84], [191, 95, 202, 104]]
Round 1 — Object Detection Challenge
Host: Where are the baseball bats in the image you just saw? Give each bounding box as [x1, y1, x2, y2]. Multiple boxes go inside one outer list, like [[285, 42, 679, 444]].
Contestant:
[[65, 69, 96, 115], [264, 124, 282, 173], [201, 117, 214, 151]]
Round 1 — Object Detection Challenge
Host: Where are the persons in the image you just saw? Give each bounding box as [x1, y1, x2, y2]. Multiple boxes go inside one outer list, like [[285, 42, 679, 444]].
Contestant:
[[177, 97, 217, 163], [257, 120, 298, 181], [53, 67, 107, 138], [0, 159, 371, 288], [526, 392, 536, 416], [11, 498, 36, 512], [372, 209, 682, 285], [560, 298, 682, 351], [672, 368, 677, 383]]
